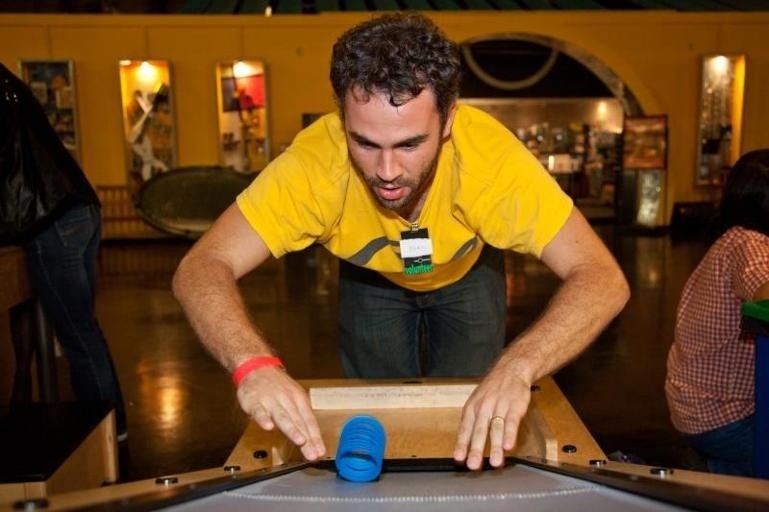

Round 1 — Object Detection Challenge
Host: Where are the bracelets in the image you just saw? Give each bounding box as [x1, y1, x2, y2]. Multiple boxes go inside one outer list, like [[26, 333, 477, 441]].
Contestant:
[[229, 354, 290, 390]]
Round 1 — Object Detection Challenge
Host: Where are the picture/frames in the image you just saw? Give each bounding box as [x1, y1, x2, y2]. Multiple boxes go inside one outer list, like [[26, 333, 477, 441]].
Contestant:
[[19, 55, 82, 173]]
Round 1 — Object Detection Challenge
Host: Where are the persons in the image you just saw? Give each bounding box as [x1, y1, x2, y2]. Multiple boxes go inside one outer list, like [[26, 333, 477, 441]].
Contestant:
[[661, 146, 769, 474], [169, 9, 634, 472], [1, 62, 132, 444]]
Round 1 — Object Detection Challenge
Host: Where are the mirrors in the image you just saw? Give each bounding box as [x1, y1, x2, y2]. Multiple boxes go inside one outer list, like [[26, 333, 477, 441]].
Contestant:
[[115, 53, 273, 207]]
